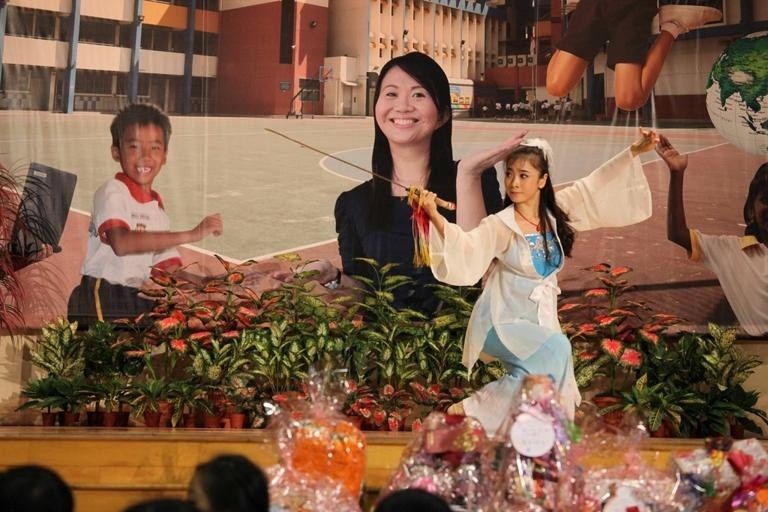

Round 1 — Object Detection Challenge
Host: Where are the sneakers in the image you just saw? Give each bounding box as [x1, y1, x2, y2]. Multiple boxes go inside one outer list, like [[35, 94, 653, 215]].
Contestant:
[[658, 2, 722, 33]]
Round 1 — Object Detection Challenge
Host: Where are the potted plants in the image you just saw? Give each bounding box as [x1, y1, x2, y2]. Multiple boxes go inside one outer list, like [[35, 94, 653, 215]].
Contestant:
[[13, 252, 768, 439]]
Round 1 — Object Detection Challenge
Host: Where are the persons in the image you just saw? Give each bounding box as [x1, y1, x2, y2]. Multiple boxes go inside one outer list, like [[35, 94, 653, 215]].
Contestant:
[[0, 464, 78, 512], [62, 103, 224, 330], [655, 133, 768, 342], [188, 455, 270, 512], [529, 37, 536, 57], [481, 93, 573, 125], [270, 51, 505, 319], [547, 1, 722, 109], [413, 127, 659, 440], [120, 500, 201, 511]]
